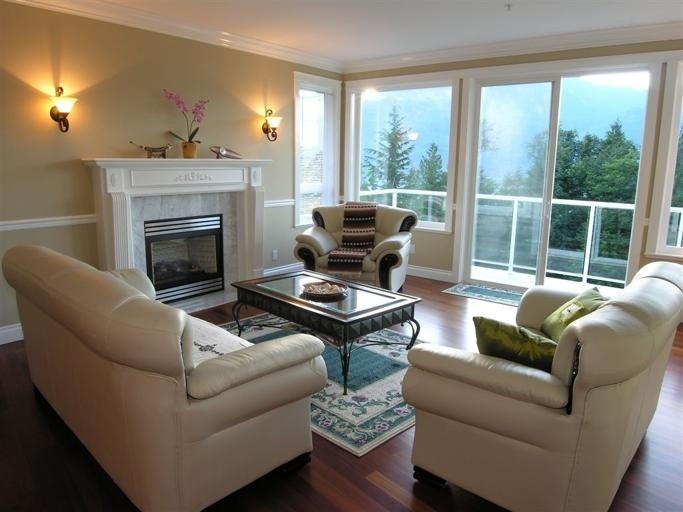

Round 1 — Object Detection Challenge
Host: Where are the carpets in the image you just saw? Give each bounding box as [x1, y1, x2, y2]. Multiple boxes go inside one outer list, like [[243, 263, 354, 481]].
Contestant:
[[217, 312, 430, 457], [441, 283, 523, 307]]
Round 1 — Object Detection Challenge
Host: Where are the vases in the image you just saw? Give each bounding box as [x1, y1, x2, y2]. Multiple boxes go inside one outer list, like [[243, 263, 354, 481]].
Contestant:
[[181, 142, 197, 159]]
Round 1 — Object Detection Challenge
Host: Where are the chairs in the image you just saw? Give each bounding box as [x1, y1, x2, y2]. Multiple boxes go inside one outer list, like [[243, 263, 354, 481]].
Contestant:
[[294, 201, 418, 292], [402, 261, 683, 512]]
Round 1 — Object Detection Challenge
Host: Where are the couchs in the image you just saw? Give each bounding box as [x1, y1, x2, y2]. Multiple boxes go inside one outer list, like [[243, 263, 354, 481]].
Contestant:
[[1, 245, 328, 512]]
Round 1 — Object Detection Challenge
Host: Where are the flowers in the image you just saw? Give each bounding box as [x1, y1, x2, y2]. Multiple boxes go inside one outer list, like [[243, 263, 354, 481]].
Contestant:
[[162, 88, 210, 143]]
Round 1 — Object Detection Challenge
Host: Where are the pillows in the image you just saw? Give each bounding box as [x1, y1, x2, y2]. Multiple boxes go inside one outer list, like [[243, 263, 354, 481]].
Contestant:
[[539, 287, 609, 342], [473, 316, 557, 372]]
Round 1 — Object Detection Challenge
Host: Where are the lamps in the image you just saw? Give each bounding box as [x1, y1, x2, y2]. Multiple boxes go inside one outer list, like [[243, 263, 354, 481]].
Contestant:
[[262, 110, 282, 141], [50, 88, 78, 133]]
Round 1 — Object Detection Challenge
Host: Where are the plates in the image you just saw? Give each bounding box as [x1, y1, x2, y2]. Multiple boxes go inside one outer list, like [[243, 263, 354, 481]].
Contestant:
[[301, 281, 348, 299]]
